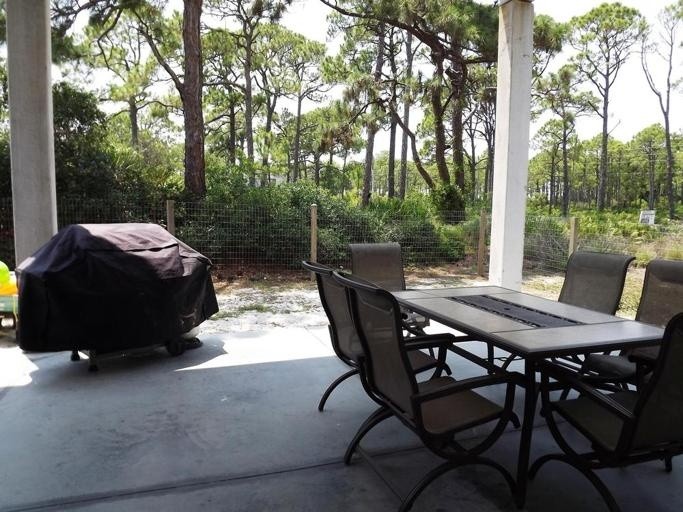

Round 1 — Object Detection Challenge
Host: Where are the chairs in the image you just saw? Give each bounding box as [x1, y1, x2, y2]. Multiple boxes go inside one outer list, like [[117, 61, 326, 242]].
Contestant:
[[576, 259, 683, 409], [529, 309, 681, 511], [302, 258, 454, 451], [500, 250, 636, 418], [330, 269, 522, 510], [348, 242, 435, 361]]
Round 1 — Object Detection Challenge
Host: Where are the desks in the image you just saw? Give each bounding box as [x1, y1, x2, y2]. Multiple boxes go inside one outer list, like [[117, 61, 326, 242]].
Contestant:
[[383, 284, 665, 510]]
